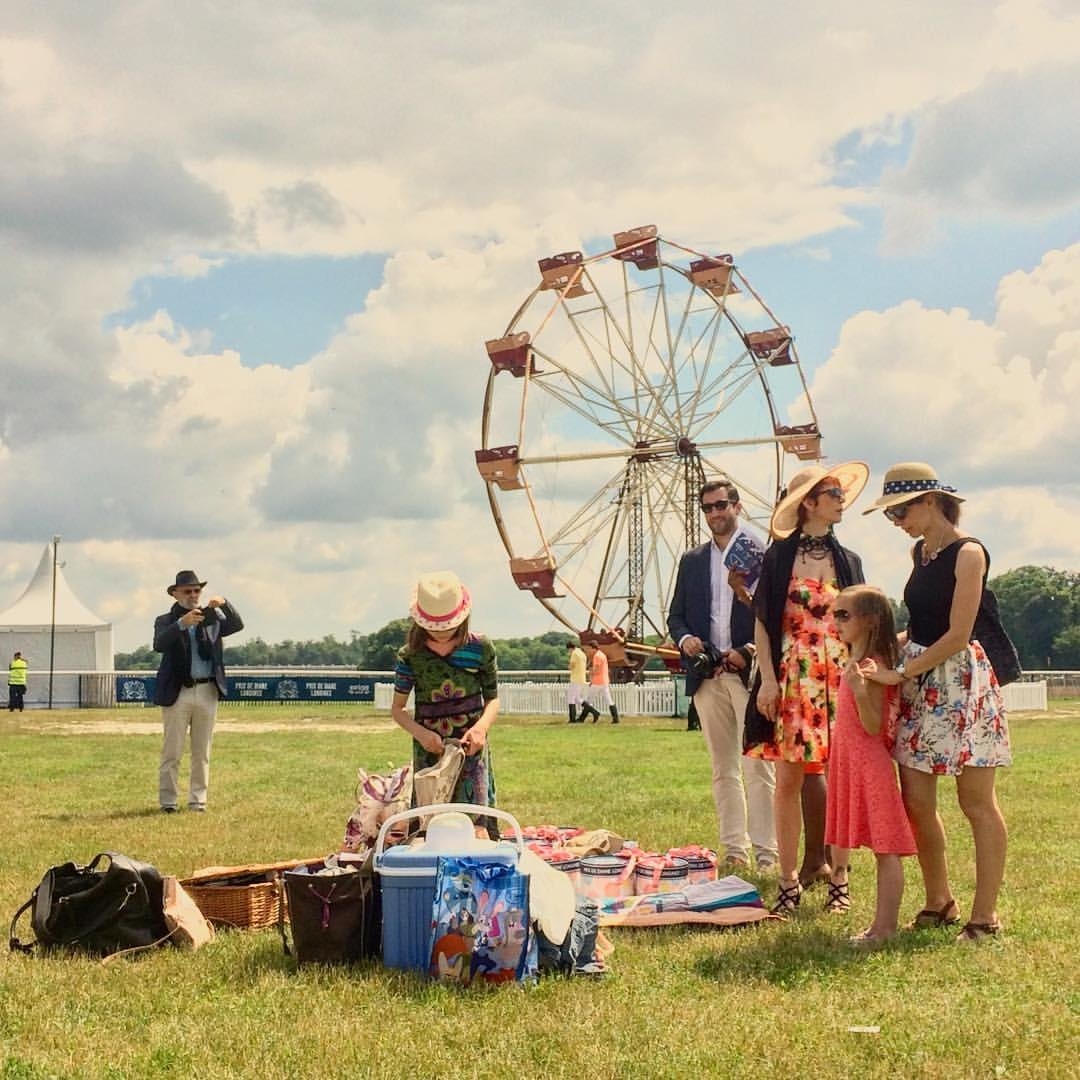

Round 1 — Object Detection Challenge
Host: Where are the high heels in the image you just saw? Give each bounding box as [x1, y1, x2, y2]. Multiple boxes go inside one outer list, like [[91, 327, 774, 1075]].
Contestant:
[[771, 882, 803, 915], [822, 881, 850, 917]]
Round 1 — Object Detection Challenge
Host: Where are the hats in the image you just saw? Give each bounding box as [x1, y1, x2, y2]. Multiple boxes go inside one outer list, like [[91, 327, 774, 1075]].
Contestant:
[[167, 570, 208, 596], [861, 462, 966, 516], [770, 460, 870, 540], [409, 570, 470, 632]]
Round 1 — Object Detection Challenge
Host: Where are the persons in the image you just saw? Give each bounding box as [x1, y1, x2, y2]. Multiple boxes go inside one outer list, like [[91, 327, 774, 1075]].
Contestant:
[[391, 571, 501, 845], [566, 641, 600, 724], [825, 585, 918, 946], [668, 481, 778, 872], [577, 640, 619, 725], [8, 652, 27, 712], [755, 462, 871, 918], [862, 462, 1009, 943], [728, 487, 832, 889], [153, 571, 244, 813]]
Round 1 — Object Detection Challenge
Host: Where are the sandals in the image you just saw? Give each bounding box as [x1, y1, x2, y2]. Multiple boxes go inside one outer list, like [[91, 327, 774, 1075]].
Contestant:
[[906, 899, 961, 930], [954, 920, 999, 940]]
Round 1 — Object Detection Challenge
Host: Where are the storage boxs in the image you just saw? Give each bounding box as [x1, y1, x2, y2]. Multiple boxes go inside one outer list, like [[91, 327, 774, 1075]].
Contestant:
[[373, 803, 523, 974]]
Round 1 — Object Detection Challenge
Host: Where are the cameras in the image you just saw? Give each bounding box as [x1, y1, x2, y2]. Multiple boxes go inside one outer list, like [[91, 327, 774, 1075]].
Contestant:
[[694, 640, 723, 679]]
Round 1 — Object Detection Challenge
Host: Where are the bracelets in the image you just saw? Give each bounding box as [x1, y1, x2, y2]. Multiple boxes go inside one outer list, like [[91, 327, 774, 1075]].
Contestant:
[[738, 592, 750, 602]]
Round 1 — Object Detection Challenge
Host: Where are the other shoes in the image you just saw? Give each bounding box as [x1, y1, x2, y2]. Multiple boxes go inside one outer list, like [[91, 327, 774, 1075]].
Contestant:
[[726, 856, 744, 869], [797, 862, 833, 888], [847, 920, 898, 948]]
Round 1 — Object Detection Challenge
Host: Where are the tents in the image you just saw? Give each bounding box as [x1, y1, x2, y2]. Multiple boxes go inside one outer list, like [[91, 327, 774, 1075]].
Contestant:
[[0, 544, 114, 708]]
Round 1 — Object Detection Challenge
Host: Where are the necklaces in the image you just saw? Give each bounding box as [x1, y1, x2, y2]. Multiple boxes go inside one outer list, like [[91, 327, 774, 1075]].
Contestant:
[[922, 517, 947, 567], [797, 531, 835, 566]]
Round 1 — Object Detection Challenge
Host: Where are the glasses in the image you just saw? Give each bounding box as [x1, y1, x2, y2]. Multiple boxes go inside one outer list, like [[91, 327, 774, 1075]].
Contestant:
[[883, 498, 922, 522], [813, 487, 850, 498], [832, 608, 852, 622], [175, 589, 200, 595], [700, 499, 736, 513]]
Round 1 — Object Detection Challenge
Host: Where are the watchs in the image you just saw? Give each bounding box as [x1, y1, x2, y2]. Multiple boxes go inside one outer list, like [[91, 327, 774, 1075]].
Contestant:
[[895, 664, 911, 680]]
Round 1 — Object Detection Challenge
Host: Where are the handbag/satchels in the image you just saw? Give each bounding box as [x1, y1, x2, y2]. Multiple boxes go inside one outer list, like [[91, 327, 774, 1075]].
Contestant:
[[520, 847, 576, 946], [9, 852, 163, 955], [343, 760, 413, 853], [414, 738, 470, 832], [428, 856, 530, 989], [163, 875, 219, 950], [284, 861, 382, 969], [972, 589, 1023, 689]]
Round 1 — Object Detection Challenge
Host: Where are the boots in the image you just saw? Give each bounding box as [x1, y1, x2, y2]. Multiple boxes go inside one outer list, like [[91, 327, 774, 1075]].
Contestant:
[[568, 703, 577, 724], [582, 701, 599, 722], [574, 707, 589, 723], [610, 704, 619, 724]]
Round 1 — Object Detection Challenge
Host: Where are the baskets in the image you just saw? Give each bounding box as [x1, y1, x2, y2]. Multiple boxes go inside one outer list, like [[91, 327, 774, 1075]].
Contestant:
[[181, 880, 289, 927]]
